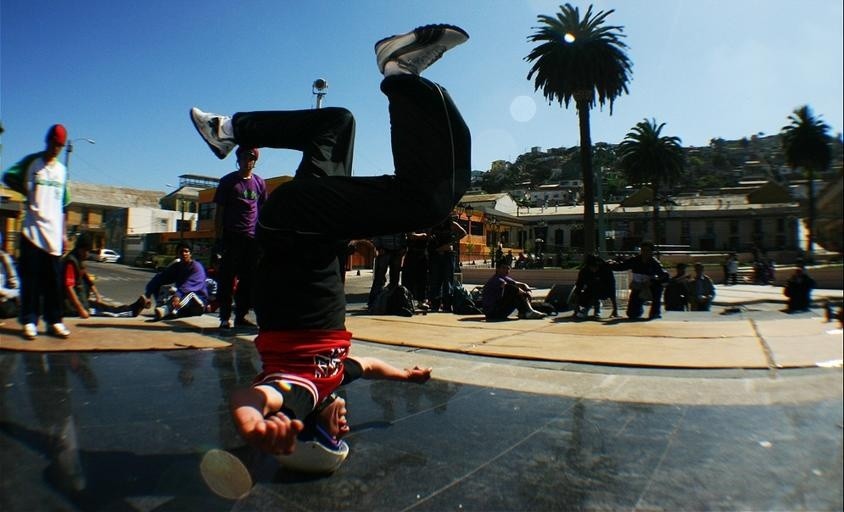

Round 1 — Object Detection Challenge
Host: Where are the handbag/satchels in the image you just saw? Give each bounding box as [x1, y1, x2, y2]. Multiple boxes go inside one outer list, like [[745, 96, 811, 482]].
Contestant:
[[372, 285, 415, 317], [451, 285, 485, 314]]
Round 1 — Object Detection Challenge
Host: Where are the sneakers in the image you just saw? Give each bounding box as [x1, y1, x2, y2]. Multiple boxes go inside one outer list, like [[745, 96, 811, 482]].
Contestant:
[[220, 321, 231, 331], [375, 24, 469, 74], [519, 310, 547, 319], [157, 306, 169, 320], [189, 108, 236, 159], [234, 319, 258, 329], [23, 324, 37, 338], [47, 323, 70, 337]]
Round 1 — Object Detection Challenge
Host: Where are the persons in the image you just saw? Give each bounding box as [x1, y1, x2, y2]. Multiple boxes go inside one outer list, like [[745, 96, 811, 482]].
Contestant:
[[784, 270, 817, 310], [189, 22, 472, 478], [721, 248, 775, 284], [143, 243, 209, 318], [1, 250, 21, 317], [611, 243, 715, 318], [61, 235, 146, 318], [368, 218, 615, 320], [0, 123, 74, 343]]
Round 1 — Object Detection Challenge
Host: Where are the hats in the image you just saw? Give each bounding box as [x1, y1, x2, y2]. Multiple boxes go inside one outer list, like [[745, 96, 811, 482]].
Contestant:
[[46, 123, 66, 145]]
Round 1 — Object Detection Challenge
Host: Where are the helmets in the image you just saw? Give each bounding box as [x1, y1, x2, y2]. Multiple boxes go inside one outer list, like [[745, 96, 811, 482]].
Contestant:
[[271, 424, 350, 474], [233, 144, 258, 159]]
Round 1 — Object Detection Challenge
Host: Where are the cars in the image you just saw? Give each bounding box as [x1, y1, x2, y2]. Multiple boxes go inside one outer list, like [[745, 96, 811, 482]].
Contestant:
[[87, 248, 100, 260], [97, 249, 121, 263]]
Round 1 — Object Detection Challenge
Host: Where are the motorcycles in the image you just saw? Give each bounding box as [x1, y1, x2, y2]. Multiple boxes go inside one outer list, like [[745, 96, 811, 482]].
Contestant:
[[512, 256, 545, 270]]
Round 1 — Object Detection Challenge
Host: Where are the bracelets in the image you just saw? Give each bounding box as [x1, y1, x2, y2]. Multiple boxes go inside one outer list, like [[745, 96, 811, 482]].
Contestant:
[[402, 368, 413, 383]]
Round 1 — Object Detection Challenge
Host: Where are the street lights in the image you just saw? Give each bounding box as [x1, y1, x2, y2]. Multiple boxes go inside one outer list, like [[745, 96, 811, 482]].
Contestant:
[[465, 204, 475, 267], [64, 134, 99, 179], [308, 76, 329, 110], [456, 203, 466, 267]]
